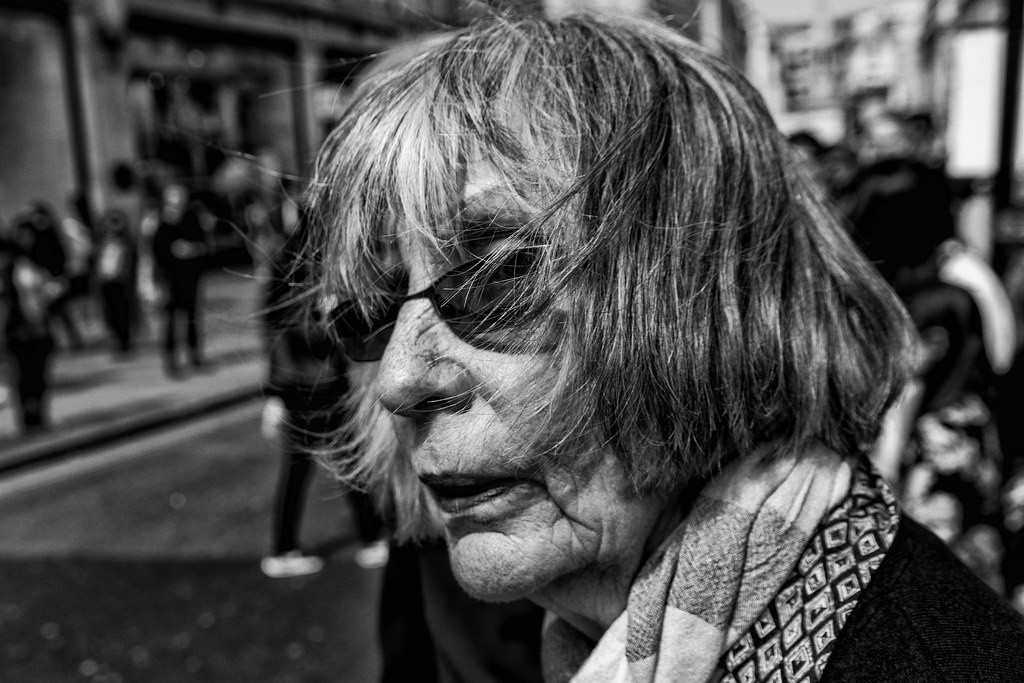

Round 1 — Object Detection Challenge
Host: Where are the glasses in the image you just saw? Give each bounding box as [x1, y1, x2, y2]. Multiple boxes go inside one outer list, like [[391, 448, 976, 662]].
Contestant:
[[326, 242, 569, 363]]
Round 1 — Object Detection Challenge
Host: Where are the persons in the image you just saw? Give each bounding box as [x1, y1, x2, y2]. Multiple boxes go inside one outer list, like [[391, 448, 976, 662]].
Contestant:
[[784, 110, 1023, 612], [297, 14, 1024, 683], [1, 149, 401, 577]]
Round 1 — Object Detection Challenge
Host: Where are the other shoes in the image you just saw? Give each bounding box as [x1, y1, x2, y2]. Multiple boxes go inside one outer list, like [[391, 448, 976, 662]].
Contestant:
[[354, 540, 391, 569], [260, 553, 322, 578]]
